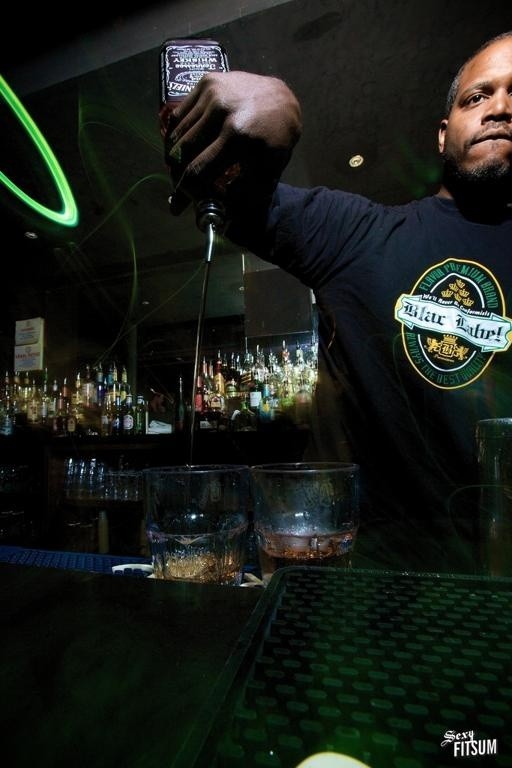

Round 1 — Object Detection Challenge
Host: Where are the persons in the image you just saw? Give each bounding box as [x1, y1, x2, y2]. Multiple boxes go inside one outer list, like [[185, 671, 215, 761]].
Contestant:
[[159, 34, 511, 555]]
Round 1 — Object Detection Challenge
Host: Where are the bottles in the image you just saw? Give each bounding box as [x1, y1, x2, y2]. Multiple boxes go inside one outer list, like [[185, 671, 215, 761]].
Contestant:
[[63, 456, 105, 485], [160, 35, 229, 235], [195, 341, 317, 431], [174, 376, 187, 434], [0, 361, 145, 438]]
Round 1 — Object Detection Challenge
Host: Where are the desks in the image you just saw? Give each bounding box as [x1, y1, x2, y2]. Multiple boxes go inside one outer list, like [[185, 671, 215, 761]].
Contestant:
[[1, 561, 265, 767]]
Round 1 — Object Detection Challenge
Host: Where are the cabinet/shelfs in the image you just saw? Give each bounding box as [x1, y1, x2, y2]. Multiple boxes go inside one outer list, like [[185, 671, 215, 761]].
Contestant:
[[0, 422, 310, 510]]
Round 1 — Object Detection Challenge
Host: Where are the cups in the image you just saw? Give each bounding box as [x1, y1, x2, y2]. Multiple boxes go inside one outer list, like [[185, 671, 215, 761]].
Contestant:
[[251, 463, 360, 589], [142, 465, 249, 587]]
